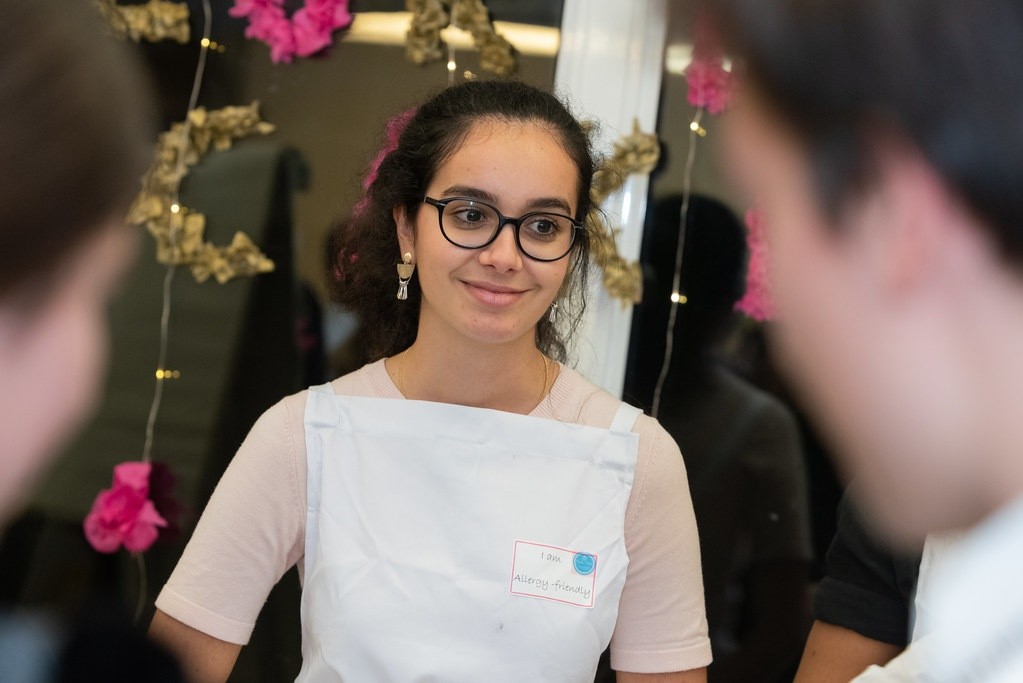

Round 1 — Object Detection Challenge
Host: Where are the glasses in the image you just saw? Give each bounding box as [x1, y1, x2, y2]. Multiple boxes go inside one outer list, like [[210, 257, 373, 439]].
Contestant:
[[415, 191, 588, 266]]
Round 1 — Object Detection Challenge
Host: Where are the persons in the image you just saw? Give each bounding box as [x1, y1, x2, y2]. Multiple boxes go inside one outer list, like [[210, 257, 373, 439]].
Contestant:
[[1, 0, 151, 683], [148, 80, 713, 683], [659, 0, 1023, 683]]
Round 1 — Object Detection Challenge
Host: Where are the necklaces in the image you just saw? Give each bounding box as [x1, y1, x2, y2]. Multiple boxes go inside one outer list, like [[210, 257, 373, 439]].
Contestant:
[[399, 348, 549, 406]]
